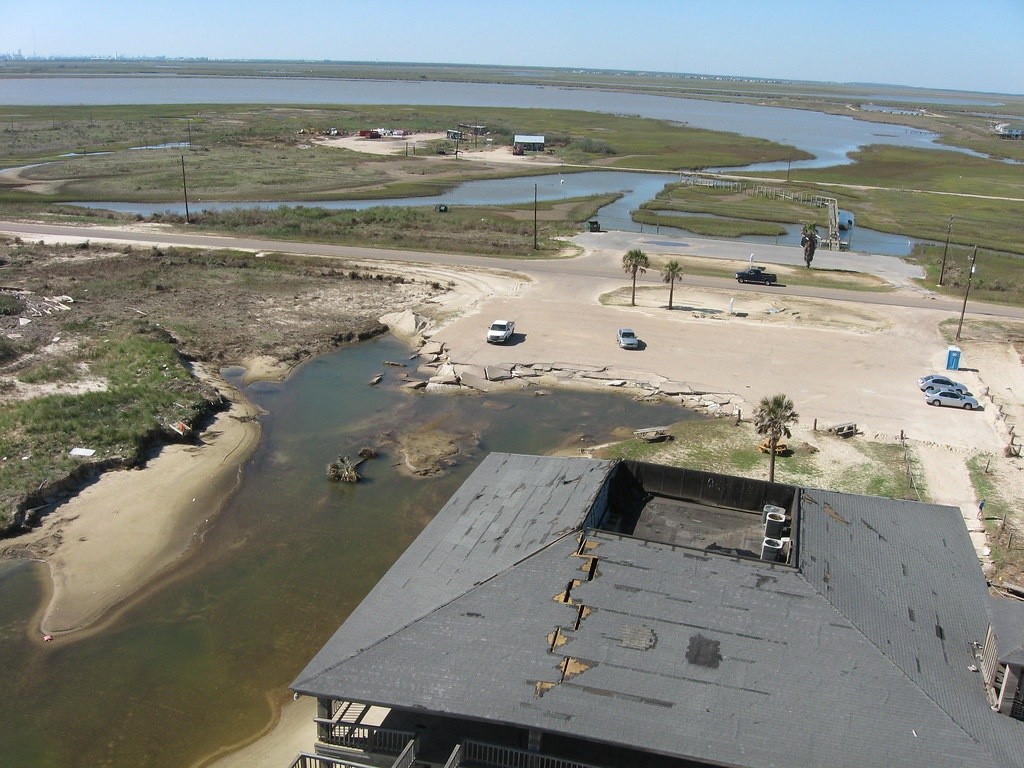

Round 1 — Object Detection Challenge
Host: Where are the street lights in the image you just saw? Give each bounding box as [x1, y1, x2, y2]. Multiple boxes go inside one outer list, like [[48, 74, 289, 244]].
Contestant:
[[749, 253, 755, 268]]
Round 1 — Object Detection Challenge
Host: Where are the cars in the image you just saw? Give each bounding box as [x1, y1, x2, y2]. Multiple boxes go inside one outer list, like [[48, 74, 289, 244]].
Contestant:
[[919, 375, 969, 394], [926, 388, 980, 409]]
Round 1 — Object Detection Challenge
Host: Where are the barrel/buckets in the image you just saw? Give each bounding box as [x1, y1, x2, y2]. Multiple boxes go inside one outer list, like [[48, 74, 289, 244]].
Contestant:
[[760, 537, 783, 562], [762, 504, 785, 539]]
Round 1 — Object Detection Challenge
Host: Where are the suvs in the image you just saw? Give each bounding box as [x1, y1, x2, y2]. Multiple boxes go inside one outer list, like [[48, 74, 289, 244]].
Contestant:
[[618, 329, 639, 350]]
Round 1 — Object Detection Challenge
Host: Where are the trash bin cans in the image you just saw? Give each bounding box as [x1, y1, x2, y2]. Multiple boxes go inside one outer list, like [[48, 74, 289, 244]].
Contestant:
[[760, 537, 784, 562], [587, 221, 600, 233], [762, 504, 786, 539], [945, 346, 961, 370]]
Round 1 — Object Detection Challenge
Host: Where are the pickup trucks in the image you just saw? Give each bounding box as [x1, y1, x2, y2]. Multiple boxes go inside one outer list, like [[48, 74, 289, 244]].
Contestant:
[[486, 319, 515, 343], [736, 269, 777, 286]]
